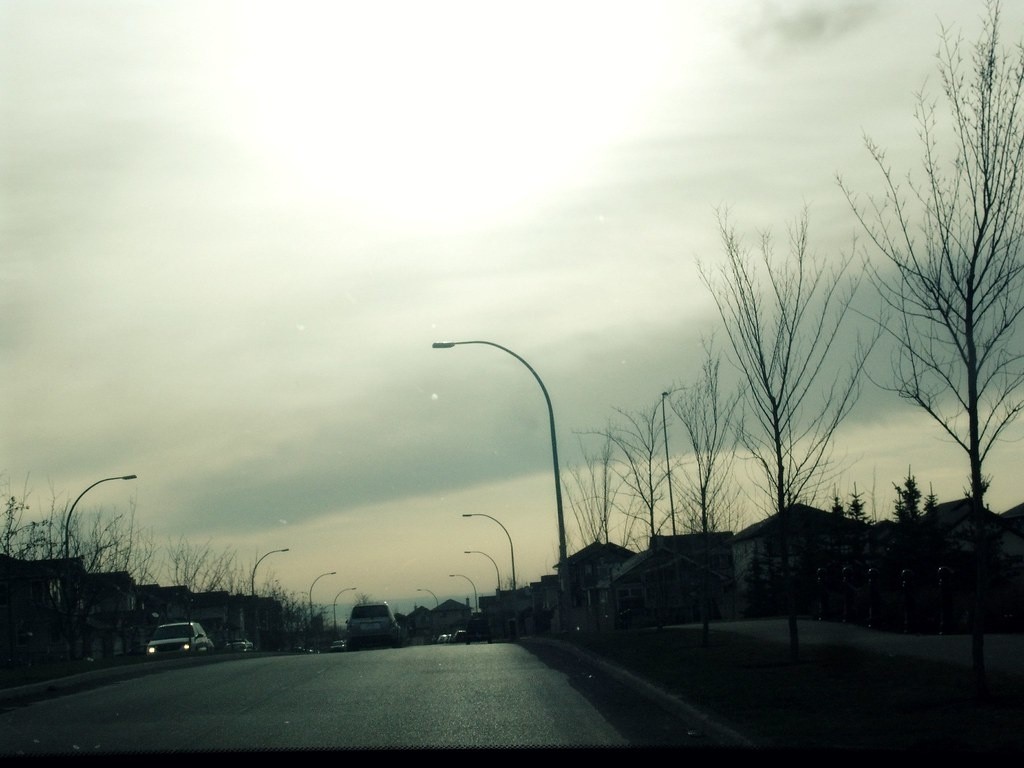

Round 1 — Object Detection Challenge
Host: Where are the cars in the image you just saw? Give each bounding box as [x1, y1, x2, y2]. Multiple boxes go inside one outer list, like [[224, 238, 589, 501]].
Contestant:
[[225, 639, 253, 652], [433, 630, 466, 644], [295, 646, 319, 653]]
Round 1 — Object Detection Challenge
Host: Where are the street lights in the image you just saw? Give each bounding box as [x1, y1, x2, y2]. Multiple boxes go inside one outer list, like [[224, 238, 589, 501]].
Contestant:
[[251, 549, 288, 597], [310, 572, 336, 618], [462, 513, 520, 638], [433, 341, 575, 632], [465, 551, 501, 607], [449, 574, 478, 615], [66, 475, 137, 610], [333, 587, 356, 629], [662, 392, 676, 549], [418, 589, 441, 623]]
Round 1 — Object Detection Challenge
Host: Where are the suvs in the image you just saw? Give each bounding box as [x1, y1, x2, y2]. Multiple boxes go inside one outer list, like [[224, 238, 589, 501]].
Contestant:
[[347, 603, 401, 651], [330, 640, 346, 652], [146, 621, 212, 658]]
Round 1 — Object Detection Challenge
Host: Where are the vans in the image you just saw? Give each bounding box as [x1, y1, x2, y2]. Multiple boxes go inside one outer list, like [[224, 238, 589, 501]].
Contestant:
[[465, 614, 492, 644]]
